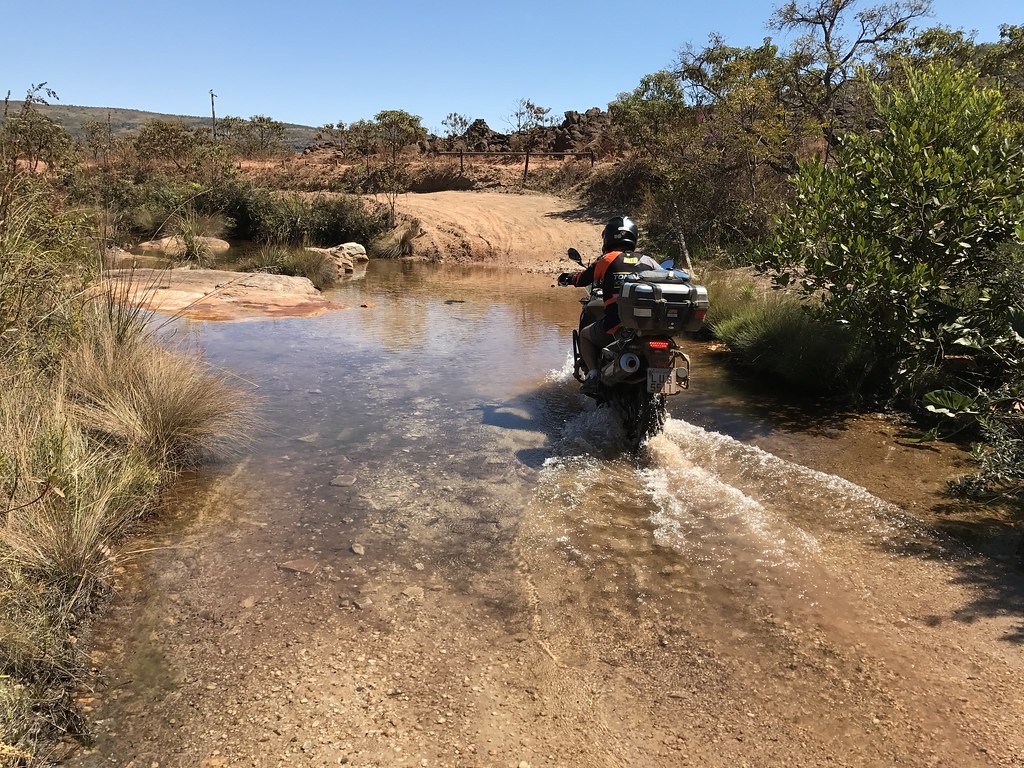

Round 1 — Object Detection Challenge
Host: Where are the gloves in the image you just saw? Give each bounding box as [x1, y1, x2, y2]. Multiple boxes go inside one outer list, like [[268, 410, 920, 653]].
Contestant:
[[558, 273, 569, 286]]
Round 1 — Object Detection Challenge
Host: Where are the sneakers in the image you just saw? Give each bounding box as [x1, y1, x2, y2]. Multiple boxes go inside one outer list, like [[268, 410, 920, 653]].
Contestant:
[[583, 370, 598, 387]]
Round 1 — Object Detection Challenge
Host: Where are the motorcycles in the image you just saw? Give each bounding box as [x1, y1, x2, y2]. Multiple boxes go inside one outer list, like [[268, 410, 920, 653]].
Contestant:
[[561, 247, 709, 447]]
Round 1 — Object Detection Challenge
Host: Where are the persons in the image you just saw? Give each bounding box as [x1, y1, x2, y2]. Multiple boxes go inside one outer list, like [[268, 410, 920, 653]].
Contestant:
[[557, 216, 666, 394]]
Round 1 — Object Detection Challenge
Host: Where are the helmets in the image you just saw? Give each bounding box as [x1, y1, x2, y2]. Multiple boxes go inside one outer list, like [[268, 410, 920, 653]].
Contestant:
[[601, 215, 638, 254]]
